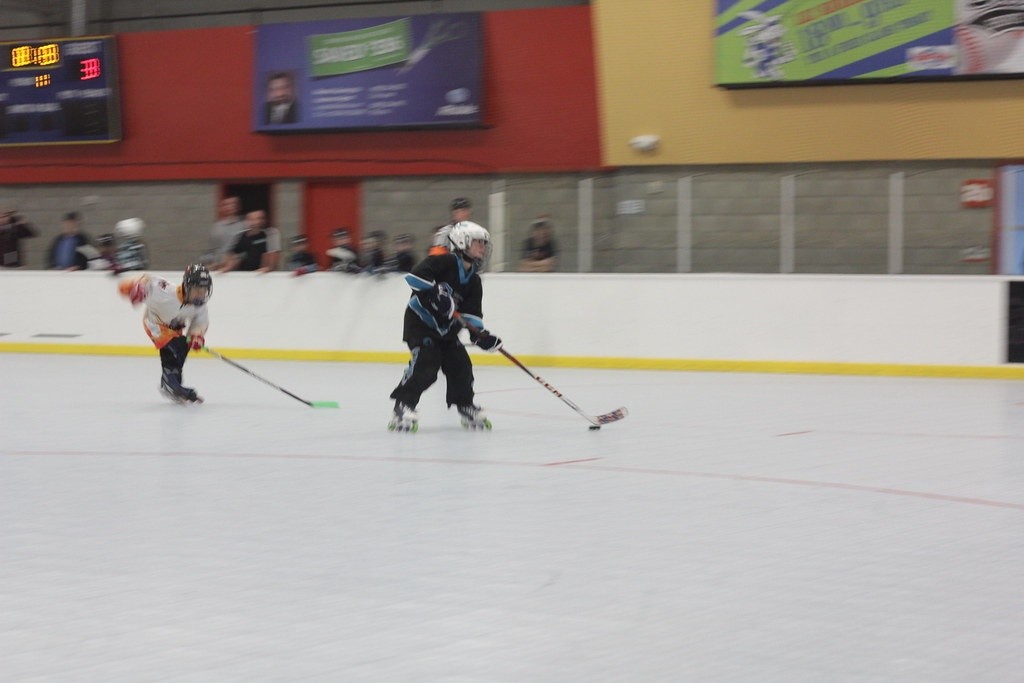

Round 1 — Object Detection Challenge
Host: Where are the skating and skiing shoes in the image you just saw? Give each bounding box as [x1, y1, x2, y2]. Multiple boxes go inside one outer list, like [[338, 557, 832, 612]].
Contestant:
[[387, 399, 419, 433], [457, 401, 492, 430]]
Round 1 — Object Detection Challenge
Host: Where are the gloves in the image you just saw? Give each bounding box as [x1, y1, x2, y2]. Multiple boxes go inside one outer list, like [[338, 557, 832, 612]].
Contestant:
[[430, 283, 455, 319], [469, 328, 503, 352], [187, 332, 204, 350], [129, 284, 144, 304]]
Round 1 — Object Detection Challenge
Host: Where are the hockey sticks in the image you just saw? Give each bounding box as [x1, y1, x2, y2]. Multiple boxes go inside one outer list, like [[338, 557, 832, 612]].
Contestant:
[[452, 311, 629, 426], [202, 346, 339, 408]]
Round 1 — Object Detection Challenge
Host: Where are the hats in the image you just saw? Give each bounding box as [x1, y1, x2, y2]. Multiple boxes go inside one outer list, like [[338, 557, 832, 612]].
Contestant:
[[452, 199, 472, 211]]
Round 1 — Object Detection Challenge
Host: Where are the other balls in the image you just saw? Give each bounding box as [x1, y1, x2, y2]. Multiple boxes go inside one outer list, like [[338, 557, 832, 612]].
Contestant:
[[589, 426, 601, 430]]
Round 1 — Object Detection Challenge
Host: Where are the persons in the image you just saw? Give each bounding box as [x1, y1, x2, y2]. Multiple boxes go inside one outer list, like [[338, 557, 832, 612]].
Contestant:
[[120, 264, 213, 402], [0, 209, 149, 275], [260, 73, 301, 127], [390, 222, 502, 423], [202, 196, 560, 273]]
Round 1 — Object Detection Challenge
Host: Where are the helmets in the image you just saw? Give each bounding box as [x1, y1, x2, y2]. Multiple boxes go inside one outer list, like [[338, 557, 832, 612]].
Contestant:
[[183, 263, 212, 306], [449, 221, 489, 265], [116, 217, 145, 240]]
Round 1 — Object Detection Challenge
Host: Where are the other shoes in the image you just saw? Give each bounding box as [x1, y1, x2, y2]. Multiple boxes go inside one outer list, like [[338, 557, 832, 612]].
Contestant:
[[159, 379, 203, 405]]
